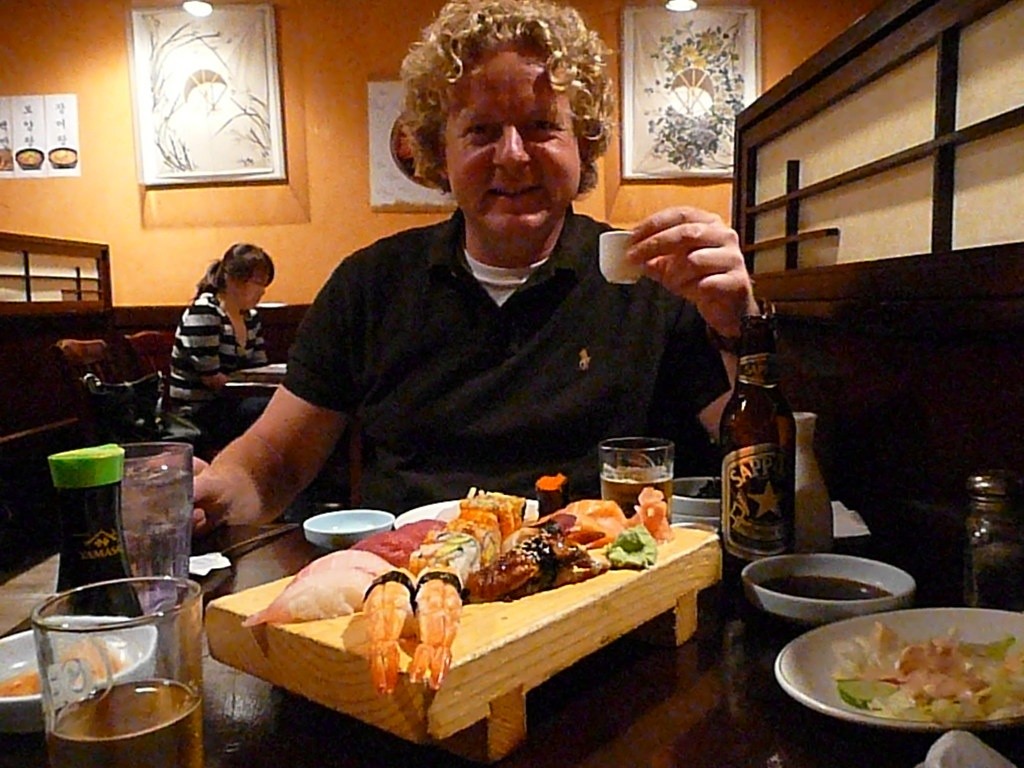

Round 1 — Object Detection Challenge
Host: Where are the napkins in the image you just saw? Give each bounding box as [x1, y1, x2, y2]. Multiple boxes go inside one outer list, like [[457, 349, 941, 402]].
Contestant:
[[830, 501, 871, 538]]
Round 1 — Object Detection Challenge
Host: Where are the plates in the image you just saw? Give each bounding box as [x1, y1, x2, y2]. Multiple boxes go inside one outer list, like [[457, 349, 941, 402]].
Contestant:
[[394, 498, 539, 530], [669, 476, 721, 526], [773, 606, 1024, 732], [0, 614, 157, 733], [742, 553, 917, 622], [303, 508, 396, 550]]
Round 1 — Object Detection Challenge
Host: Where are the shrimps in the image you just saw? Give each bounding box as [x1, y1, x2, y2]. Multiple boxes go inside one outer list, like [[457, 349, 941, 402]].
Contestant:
[[363, 564, 470, 695]]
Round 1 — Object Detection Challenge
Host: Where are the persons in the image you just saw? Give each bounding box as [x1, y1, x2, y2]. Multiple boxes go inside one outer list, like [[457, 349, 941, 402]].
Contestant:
[[167, 243, 278, 436], [134, 0, 790, 530]]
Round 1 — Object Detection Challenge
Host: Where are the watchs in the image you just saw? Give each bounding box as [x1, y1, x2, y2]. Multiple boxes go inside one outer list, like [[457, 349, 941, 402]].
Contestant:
[[707, 299, 779, 356]]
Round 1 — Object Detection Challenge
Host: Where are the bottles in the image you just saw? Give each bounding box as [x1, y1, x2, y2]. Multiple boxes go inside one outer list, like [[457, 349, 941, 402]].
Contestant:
[[718, 316, 797, 587], [794, 411, 834, 553], [959, 470, 1024, 611], [46, 444, 145, 621]]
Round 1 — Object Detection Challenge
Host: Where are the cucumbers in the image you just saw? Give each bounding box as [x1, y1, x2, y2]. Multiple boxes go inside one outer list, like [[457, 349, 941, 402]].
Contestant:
[[839, 681, 898, 711]]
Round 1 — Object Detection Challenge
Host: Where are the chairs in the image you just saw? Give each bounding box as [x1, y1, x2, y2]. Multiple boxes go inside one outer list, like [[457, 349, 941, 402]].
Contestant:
[[49, 331, 231, 465]]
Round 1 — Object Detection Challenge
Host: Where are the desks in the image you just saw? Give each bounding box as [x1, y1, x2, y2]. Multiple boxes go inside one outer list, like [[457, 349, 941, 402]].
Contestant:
[[226, 374, 365, 510]]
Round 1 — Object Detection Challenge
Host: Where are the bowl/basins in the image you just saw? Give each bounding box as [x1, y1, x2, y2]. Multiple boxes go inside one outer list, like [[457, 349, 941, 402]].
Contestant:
[[15, 148, 45, 169], [48, 148, 78, 168]]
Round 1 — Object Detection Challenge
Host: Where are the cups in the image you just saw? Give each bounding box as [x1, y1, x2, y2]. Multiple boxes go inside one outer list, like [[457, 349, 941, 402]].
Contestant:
[[104, 442, 193, 610], [29, 575, 205, 768], [599, 437, 675, 525], [598, 230, 645, 284]]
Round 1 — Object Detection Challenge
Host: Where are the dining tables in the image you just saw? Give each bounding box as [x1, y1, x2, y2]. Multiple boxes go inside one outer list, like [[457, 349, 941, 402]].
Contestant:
[[0, 524, 1024, 768]]
